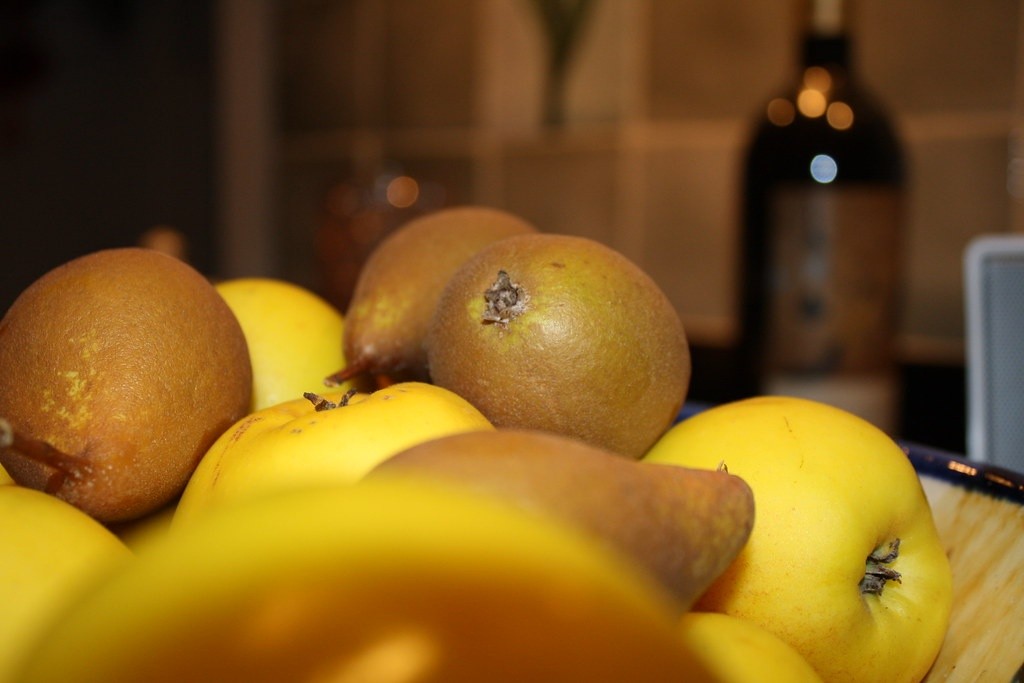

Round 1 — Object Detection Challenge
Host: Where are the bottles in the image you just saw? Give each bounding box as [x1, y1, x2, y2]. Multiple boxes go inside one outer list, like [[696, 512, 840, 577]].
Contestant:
[[736, 0, 907, 397]]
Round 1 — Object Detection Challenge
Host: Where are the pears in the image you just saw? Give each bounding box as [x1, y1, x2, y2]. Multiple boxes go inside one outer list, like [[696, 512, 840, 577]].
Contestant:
[[357, 428, 755, 614], [343, 207, 691, 458], [1, 245, 253, 525]]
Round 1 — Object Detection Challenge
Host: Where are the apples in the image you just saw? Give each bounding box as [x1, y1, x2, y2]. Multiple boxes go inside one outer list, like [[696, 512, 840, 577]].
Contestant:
[[0, 278, 951, 683]]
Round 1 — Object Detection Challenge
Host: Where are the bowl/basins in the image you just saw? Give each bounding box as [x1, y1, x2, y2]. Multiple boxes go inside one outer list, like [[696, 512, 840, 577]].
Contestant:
[[674, 400, 1024, 683]]
